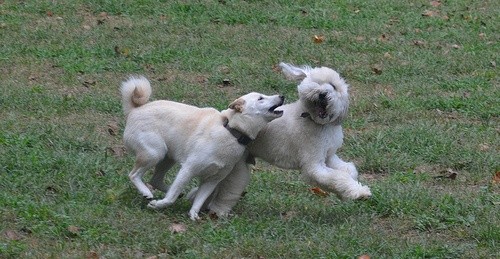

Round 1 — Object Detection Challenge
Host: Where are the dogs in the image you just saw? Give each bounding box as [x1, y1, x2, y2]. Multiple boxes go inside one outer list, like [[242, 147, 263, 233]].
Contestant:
[[186, 62, 372, 219], [120, 75, 285, 221]]
